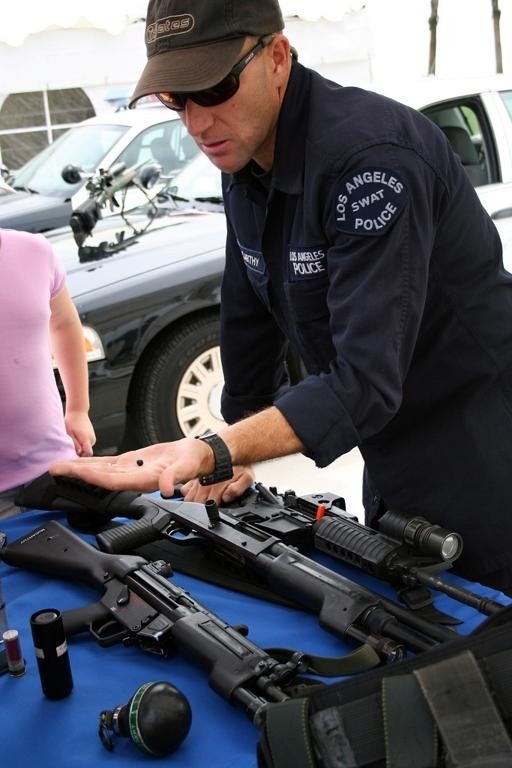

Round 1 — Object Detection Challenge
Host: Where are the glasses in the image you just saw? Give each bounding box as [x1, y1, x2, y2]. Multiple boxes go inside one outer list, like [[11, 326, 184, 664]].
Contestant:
[[155, 32, 277, 112]]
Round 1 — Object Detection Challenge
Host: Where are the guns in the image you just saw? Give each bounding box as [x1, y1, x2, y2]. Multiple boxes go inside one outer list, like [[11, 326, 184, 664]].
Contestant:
[[29, 472, 473, 668], [160, 470, 509, 626], [68, 157, 154, 247], [3, 518, 320, 731]]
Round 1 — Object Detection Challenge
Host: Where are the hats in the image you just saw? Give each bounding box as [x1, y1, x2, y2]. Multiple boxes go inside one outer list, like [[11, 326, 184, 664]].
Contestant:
[[128, 0, 286, 110]]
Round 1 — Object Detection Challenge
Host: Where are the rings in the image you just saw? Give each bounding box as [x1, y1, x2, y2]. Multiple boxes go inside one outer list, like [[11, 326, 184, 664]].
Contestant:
[[81, 438, 91, 446]]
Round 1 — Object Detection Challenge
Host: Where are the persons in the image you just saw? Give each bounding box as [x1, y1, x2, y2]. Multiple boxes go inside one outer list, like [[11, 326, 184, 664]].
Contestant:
[[44, 0, 512, 602], [0, 223, 98, 519]]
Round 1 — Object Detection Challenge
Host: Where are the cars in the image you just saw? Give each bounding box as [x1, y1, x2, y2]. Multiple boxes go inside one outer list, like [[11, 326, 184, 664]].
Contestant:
[[41, 75, 512, 455], [0, 104, 200, 230]]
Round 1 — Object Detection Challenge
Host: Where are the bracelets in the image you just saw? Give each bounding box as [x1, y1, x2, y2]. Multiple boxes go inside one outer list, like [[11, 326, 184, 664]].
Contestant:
[[192, 431, 234, 488]]
